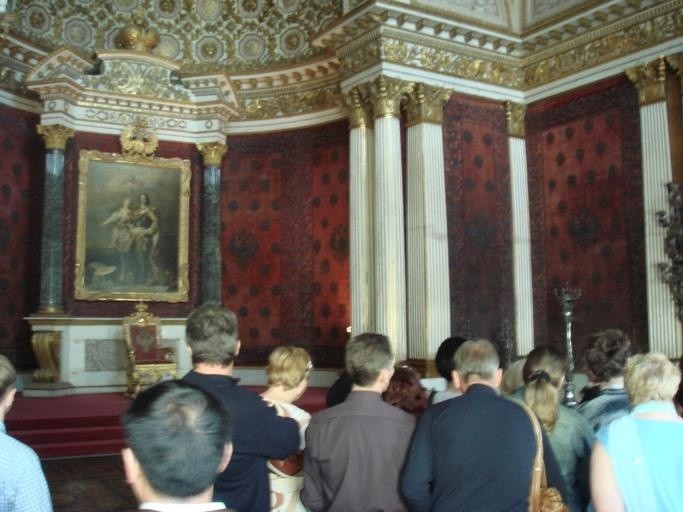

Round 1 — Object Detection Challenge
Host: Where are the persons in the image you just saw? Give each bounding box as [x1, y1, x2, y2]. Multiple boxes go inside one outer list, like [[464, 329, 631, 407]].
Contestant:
[[384, 365, 428, 416], [177, 302, 301, 512], [100, 193, 160, 285], [299, 332, 417, 511], [423, 337, 467, 406], [589, 351, 683, 512], [398, 339, 538, 512], [571, 327, 632, 431], [509, 344, 595, 512], [258, 346, 311, 511], [116, 379, 235, 512], [1, 354, 55, 512]]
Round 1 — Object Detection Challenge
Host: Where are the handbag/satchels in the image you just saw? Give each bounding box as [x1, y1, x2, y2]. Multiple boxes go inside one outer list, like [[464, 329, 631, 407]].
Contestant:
[[520, 403, 568, 512]]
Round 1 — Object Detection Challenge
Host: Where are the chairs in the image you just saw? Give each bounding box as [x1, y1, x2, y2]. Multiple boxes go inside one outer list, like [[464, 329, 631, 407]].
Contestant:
[[122, 303, 178, 399]]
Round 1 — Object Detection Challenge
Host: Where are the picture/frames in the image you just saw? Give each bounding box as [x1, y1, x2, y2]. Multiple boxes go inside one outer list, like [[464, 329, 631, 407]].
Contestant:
[[74, 148, 193, 304]]
